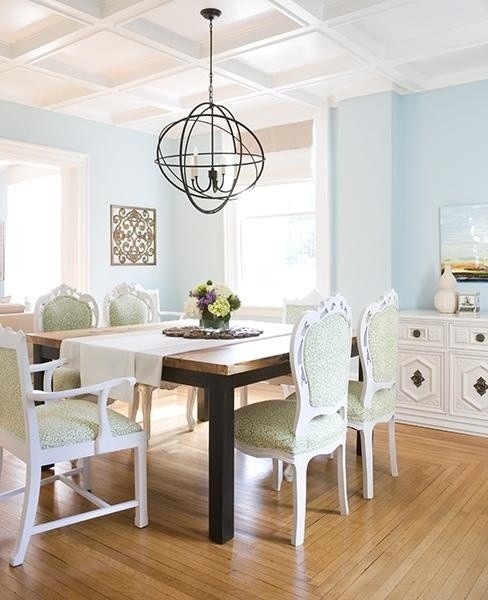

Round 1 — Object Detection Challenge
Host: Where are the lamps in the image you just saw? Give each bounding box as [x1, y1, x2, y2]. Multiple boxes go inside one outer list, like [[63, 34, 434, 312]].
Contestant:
[[153, 6, 266, 216]]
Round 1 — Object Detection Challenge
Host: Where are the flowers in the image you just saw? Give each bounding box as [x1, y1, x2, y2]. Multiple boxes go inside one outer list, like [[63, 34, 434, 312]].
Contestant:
[[184, 279, 241, 320]]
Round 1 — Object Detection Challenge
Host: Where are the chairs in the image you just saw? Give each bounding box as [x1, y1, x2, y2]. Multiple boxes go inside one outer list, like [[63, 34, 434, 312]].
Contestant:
[[0, 304, 36, 359], [102, 281, 198, 449], [0, 320, 148, 567], [239, 289, 325, 407], [234, 293, 352, 546], [35, 283, 116, 470], [284, 288, 401, 500], [134, 282, 186, 320]]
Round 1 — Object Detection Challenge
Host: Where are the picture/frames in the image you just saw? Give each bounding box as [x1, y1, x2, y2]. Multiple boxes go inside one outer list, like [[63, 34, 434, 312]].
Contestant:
[[110, 204, 157, 266]]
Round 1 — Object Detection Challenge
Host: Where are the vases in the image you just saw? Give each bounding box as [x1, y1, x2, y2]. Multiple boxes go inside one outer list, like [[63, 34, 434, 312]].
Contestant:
[[204, 319, 229, 329], [434, 265, 459, 313]]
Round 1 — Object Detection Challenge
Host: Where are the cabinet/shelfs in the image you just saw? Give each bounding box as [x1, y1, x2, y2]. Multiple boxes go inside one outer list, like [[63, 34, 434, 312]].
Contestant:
[[395, 318, 488, 439]]
